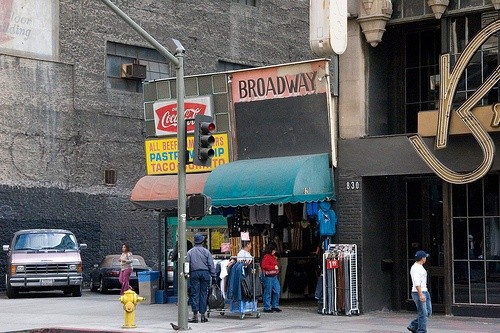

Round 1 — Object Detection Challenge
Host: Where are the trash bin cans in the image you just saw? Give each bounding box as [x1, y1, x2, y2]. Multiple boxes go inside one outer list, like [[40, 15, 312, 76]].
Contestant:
[[138, 271, 161, 303]]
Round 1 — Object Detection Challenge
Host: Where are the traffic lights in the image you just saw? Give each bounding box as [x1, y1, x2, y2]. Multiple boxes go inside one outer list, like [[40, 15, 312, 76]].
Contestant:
[[187, 193, 212, 217], [193, 112, 214, 166]]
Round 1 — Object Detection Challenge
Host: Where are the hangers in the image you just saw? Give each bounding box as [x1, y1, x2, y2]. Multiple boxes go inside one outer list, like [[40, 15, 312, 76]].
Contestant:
[[239, 259, 253, 268]]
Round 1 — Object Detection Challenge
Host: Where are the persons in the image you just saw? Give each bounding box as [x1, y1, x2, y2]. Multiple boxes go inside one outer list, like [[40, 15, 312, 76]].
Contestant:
[[118, 243, 134, 294], [407, 250, 433, 333], [171, 234, 282, 323], [468, 235, 489, 272]]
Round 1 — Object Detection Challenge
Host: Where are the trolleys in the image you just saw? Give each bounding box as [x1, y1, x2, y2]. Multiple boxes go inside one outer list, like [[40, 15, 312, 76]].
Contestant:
[[204, 256, 261, 321]]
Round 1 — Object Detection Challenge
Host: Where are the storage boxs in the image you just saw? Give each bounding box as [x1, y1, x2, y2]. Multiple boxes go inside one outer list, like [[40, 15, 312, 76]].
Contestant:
[[155, 290, 166, 304], [167, 289, 178, 303], [137, 271, 160, 304]]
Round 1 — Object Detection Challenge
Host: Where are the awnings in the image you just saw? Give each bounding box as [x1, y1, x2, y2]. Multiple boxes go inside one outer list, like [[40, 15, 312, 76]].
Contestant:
[[130, 153, 336, 211]]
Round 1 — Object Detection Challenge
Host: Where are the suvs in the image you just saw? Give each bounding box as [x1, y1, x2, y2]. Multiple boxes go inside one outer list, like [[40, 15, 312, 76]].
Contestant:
[[3, 228, 88, 299]]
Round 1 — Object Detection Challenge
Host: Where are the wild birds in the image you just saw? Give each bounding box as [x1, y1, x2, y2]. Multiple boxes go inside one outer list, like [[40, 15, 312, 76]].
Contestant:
[[170, 322, 179, 331]]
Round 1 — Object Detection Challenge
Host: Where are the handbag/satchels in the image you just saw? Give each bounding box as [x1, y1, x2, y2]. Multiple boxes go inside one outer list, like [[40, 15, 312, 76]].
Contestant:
[[207, 284, 224, 308]]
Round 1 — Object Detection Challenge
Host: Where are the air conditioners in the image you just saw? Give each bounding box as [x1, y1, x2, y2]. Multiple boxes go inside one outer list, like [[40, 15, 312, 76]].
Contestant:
[[121, 64, 147, 79]]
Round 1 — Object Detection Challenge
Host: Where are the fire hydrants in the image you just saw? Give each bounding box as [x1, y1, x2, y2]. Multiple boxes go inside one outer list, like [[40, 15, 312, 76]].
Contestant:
[[120, 288, 147, 328]]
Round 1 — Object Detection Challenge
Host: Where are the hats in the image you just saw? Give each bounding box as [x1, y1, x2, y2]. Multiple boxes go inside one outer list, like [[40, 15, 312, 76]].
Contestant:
[[415, 250, 429, 260], [193, 234, 204, 243]]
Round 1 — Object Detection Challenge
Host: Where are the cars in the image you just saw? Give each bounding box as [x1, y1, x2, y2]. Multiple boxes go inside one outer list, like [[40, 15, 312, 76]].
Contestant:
[[164, 248, 190, 289], [89, 253, 153, 295]]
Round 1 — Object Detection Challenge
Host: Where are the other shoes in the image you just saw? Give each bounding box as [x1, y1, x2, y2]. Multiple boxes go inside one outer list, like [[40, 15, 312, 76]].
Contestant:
[[188, 316, 199, 323], [200, 315, 208, 322], [263, 308, 282, 313], [407, 327, 417, 333]]
